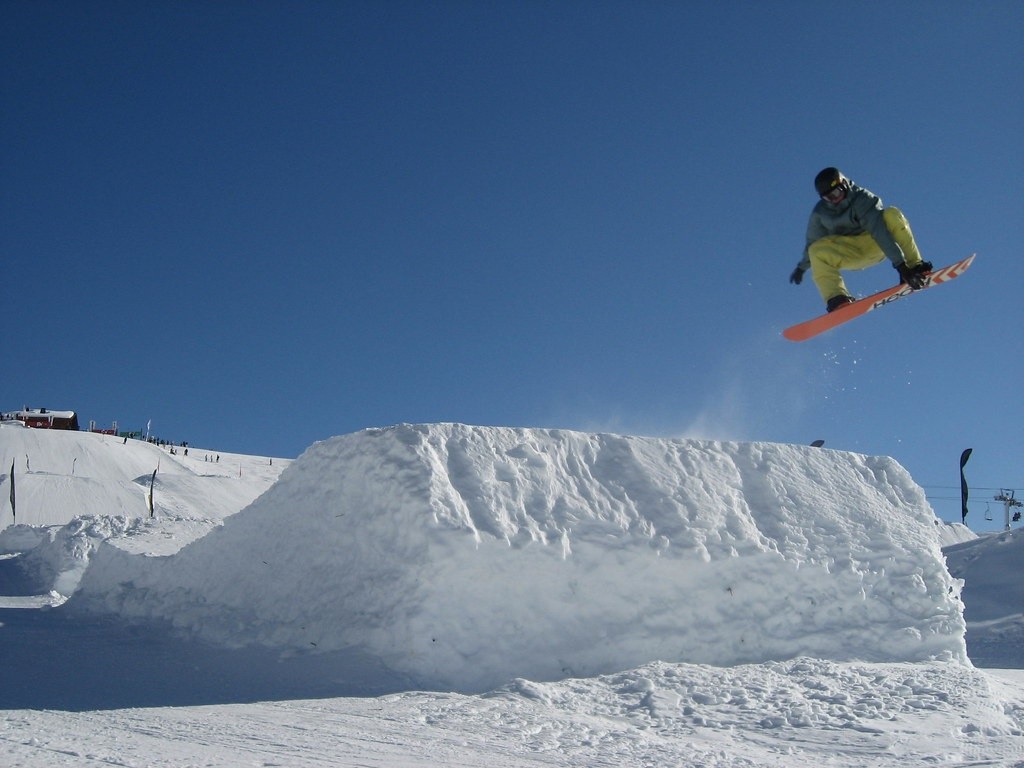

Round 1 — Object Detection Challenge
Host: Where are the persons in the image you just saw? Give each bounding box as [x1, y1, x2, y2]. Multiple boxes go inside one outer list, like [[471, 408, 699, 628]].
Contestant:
[[205, 454, 219, 462], [130, 432, 188, 455], [789, 167, 933, 312]]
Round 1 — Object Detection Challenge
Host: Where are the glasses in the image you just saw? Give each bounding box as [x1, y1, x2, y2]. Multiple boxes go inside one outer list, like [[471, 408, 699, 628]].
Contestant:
[[820, 185, 843, 203]]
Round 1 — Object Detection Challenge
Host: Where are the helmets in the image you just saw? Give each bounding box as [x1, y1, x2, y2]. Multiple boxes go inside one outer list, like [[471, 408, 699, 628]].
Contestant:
[[815, 167, 850, 193]]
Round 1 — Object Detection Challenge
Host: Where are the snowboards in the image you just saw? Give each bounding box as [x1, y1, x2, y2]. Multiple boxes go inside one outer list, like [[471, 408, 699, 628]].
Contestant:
[[782, 250, 977, 342]]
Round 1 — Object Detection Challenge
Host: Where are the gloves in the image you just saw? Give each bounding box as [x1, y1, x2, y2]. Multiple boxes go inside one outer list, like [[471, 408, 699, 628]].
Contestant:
[[790, 267, 803, 285], [897, 262, 926, 290]]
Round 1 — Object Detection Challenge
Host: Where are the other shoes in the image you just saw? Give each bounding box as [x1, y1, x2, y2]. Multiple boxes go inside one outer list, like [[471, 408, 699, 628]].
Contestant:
[[827, 295, 852, 311], [911, 260, 932, 275]]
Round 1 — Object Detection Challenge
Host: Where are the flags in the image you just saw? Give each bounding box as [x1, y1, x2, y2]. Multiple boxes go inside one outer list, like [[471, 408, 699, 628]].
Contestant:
[[10, 465, 15, 515]]
